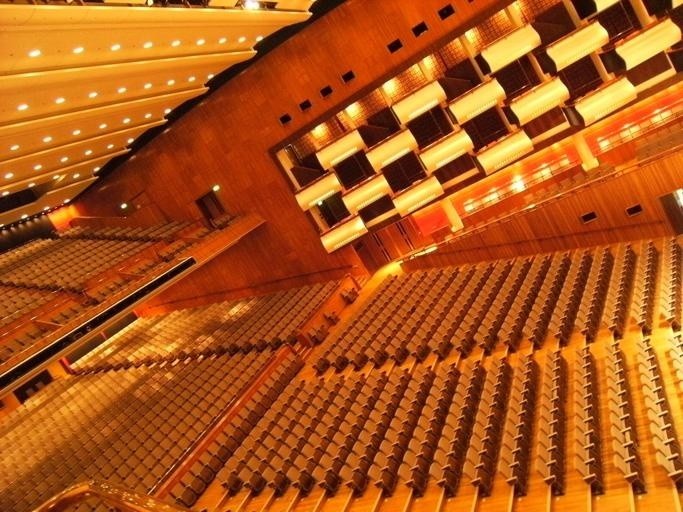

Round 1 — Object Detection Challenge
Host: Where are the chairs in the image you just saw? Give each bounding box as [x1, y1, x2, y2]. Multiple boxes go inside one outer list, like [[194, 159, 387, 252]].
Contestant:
[[0, 214, 682, 510]]
